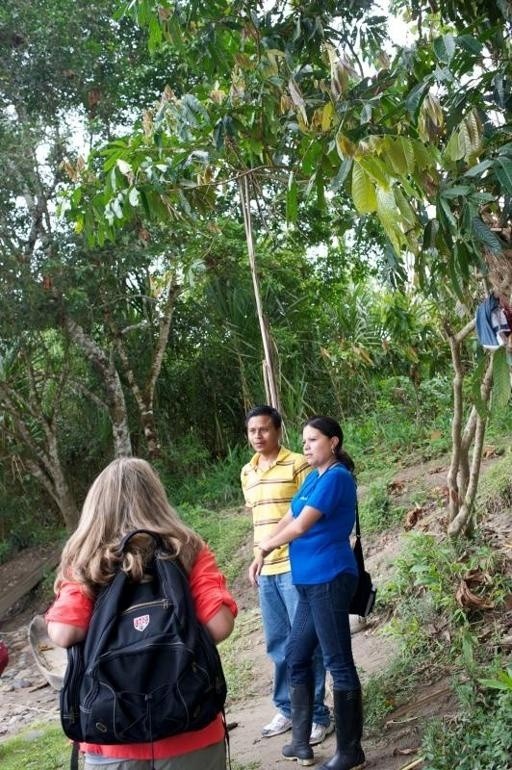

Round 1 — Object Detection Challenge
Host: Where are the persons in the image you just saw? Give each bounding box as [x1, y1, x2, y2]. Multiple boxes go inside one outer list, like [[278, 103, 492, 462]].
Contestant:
[[239, 405, 336, 748], [246, 415, 381, 770], [39, 455, 242, 770]]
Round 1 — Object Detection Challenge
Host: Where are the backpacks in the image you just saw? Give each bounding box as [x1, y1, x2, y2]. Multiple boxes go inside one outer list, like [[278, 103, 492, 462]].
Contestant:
[[60, 531, 227, 745]]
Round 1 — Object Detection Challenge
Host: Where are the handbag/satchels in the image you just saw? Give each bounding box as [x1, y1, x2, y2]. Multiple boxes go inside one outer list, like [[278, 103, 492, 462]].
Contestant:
[[352, 545, 377, 618]]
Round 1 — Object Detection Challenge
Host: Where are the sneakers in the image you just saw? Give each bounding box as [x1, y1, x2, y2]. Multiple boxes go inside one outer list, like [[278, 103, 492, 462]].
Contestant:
[[309, 722, 335, 745], [262, 713, 293, 736]]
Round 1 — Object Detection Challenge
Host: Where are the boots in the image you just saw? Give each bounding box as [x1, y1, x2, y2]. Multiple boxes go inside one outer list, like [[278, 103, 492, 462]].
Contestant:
[[281, 685, 315, 765], [317, 688, 367, 769]]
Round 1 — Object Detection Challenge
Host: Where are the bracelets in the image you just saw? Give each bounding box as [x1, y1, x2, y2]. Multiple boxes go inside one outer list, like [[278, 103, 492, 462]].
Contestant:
[[259, 546, 263, 550]]
